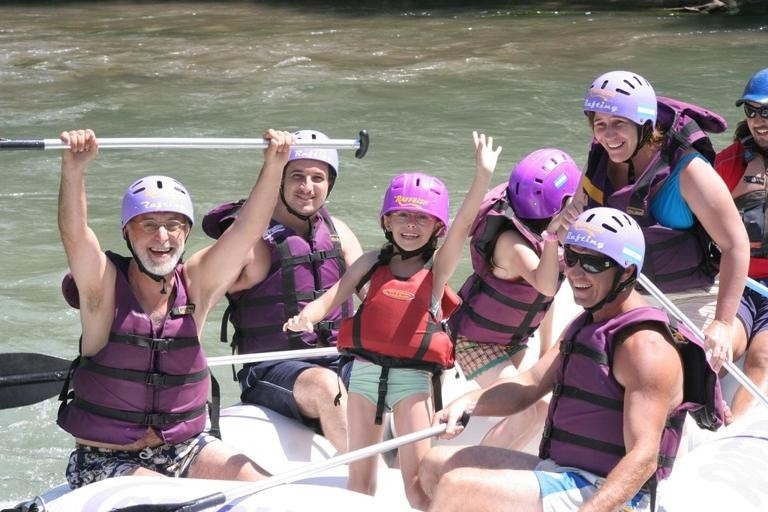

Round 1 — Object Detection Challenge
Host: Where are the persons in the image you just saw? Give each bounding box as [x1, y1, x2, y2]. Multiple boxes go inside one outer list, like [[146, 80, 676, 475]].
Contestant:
[[451, 149, 578, 388], [703, 68, 767, 424], [223, 129, 375, 455], [54, 124, 296, 512], [283, 128, 502, 512], [419, 206, 683, 512], [558, 71, 752, 374]]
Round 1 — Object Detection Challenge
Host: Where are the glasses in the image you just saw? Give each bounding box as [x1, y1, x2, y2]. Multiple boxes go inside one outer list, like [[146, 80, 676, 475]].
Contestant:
[[743, 102, 767, 119], [130, 218, 187, 237], [562, 248, 615, 274], [389, 210, 437, 228]]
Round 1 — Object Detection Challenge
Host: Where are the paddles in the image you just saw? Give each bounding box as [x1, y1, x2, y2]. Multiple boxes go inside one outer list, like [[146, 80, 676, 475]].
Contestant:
[[116, 413, 468, 511], [0, 347, 343, 411]]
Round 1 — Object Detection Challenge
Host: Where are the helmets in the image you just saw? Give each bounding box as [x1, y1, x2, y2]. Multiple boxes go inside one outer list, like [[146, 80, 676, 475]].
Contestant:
[[289, 129, 338, 177], [508, 148, 580, 220], [379, 172, 448, 238], [584, 70, 657, 133], [120, 175, 193, 229], [735, 67, 767, 106], [562, 207, 645, 280]]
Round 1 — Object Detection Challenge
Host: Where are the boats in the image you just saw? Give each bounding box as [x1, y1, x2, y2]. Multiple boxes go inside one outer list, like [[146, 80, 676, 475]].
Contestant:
[[0, 254, 768, 512]]
